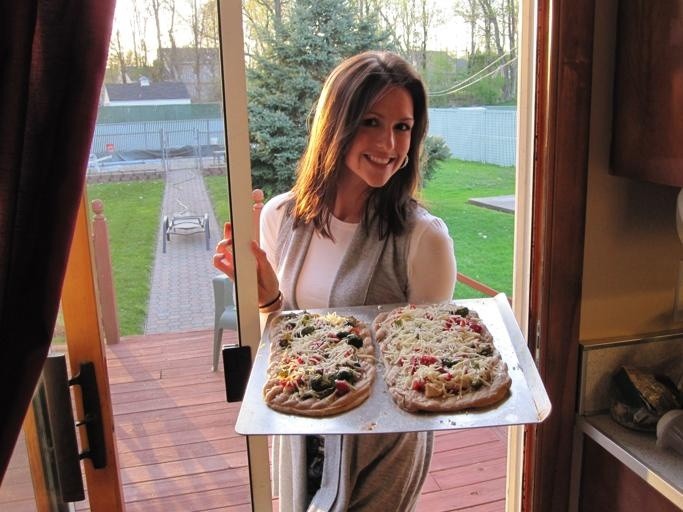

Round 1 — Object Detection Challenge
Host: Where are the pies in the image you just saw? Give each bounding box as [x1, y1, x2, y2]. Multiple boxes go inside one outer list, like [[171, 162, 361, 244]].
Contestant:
[[376, 303, 512, 413], [262, 312, 376, 417]]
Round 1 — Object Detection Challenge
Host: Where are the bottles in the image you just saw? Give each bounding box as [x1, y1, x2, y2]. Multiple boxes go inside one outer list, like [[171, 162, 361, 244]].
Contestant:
[[655, 409, 683, 459]]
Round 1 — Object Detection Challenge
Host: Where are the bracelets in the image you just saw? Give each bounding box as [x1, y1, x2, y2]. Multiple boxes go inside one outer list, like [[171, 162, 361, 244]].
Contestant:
[[259, 291, 281, 310]]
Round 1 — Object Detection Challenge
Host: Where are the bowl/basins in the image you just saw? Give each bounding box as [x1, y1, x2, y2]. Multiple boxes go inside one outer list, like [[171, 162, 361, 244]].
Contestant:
[[609, 394, 657, 433]]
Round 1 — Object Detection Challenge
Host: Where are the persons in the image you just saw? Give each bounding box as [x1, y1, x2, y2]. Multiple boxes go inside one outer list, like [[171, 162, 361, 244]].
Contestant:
[[212, 48, 461, 512]]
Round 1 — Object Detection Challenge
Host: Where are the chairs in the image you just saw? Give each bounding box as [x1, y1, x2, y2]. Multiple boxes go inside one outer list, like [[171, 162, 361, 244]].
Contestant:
[[209, 274, 240, 374]]
